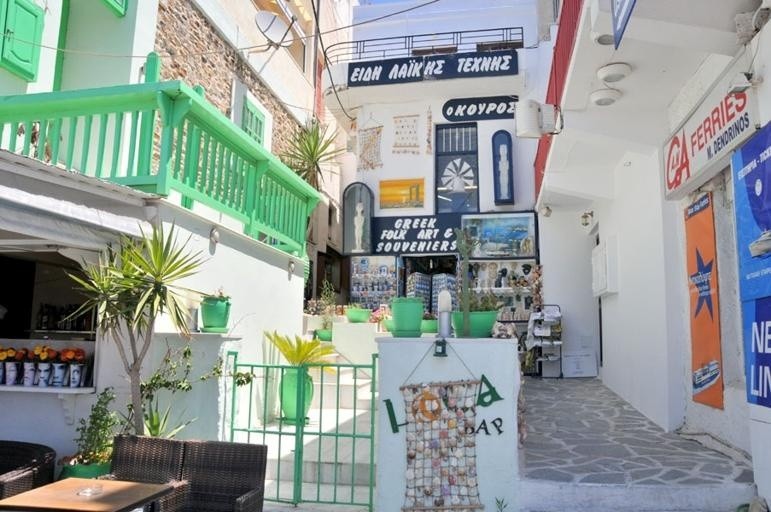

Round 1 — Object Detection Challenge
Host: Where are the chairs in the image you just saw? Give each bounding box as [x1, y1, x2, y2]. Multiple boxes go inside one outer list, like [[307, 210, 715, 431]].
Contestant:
[[0, 440, 56, 499], [152, 439, 267, 512], [93, 434, 185, 512]]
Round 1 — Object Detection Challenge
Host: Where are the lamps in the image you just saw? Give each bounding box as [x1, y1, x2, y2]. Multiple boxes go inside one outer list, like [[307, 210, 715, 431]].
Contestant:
[[587, 31, 632, 108], [513, 99, 565, 139], [437, 290, 452, 338], [185, 290, 199, 332]]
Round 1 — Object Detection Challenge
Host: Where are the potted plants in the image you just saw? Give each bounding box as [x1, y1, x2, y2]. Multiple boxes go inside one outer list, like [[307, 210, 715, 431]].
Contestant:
[[456, 289, 500, 337], [345, 308, 374, 324], [64, 344, 256, 476], [263, 327, 340, 426], [199, 286, 231, 332], [422, 310, 437, 332]]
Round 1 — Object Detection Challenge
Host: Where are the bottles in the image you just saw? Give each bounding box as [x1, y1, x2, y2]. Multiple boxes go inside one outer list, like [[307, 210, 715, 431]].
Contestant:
[[520, 276, 524, 287], [36, 302, 87, 330], [509, 271, 516, 286]]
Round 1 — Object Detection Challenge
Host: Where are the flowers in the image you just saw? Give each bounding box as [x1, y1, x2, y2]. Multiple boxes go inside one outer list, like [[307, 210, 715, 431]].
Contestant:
[[0, 345, 86, 364], [320, 306, 333, 327]]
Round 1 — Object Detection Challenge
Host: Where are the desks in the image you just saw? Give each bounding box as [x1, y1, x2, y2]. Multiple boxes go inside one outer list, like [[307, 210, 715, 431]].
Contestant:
[[0, 477, 174, 512]]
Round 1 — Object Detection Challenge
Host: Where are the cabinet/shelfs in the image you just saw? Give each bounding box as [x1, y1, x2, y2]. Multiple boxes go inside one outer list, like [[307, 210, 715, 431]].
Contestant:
[[457, 259, 541, 323]]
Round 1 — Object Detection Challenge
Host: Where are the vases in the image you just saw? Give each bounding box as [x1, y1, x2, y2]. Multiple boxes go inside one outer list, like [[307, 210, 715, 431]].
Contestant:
[[389, 296, 424, 338], [315, 328, 332, 341], [0, 361, 89, 388]]
